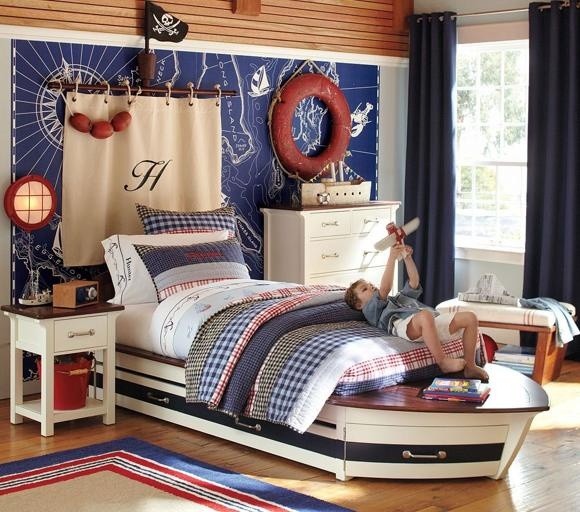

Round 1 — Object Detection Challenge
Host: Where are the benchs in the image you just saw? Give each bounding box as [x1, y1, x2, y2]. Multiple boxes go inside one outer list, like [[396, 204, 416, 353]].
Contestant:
[[145, 2, 189, 43]]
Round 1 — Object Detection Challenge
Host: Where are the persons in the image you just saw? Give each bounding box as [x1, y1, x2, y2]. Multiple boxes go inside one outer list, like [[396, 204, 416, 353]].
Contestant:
[[345, 243, 490, 381]]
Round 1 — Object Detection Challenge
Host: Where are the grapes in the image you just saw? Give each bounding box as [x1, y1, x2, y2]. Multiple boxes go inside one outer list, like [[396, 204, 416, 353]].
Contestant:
[[0, 301, 125, 437]]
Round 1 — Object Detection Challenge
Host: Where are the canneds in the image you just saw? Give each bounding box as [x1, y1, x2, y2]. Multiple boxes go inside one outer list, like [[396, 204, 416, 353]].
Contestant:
[[272, 74, 351, 180]]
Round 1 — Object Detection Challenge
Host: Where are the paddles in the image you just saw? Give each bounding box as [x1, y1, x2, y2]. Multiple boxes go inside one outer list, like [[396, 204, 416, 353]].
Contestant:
[[0, 437, 357, 512]]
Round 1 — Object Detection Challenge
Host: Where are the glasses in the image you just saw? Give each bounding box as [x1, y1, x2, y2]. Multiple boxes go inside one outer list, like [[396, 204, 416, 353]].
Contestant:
[[3, 175, 57, 306]]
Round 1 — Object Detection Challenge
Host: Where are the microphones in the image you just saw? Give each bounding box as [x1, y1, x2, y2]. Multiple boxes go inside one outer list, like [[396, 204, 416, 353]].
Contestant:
[[437, 297, 576, 386]]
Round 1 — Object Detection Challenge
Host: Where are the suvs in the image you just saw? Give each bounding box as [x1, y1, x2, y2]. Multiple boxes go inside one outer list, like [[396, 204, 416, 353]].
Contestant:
[[35, 353, 95, 410]]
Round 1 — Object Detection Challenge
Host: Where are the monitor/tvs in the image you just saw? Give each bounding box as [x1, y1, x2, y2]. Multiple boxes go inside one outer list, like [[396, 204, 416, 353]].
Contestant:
[[491, 344, 536, 375], [421, 378, 491, 403]]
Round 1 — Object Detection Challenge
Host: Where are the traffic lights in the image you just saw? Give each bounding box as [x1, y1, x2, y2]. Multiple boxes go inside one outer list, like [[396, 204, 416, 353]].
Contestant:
[[135, 203, 235, 239], [132, 237, 251, 303], [101, 231, 228, 305]]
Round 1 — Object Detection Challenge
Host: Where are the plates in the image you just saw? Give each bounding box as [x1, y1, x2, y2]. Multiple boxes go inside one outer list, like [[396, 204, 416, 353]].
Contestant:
[[260, 201, 401, 297]]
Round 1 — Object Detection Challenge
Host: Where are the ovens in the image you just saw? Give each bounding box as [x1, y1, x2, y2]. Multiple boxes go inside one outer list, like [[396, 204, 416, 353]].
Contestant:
[[86, 278, 551, 482]]
